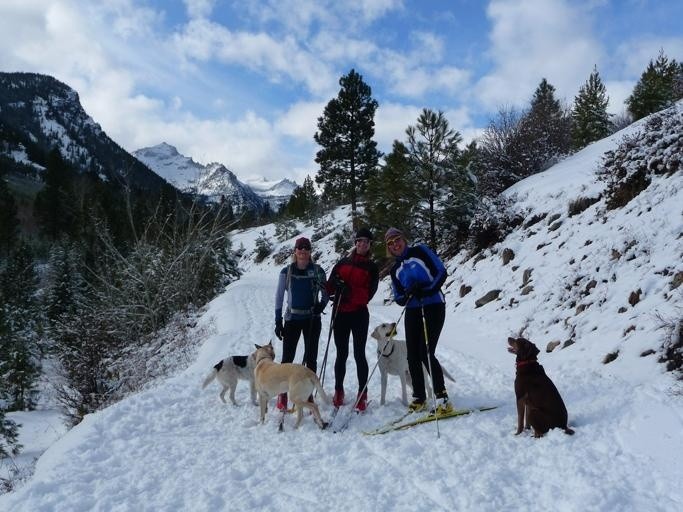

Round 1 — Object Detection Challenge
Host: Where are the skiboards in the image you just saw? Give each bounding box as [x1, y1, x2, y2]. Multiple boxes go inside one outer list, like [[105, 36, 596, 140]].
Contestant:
[[275, 399, 498, 435]]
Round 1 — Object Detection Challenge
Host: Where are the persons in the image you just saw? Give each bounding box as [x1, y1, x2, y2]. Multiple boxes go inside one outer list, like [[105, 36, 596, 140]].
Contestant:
[[323, 229, 378, 411], [274, 237, 329, 411], [384, 227, 453, 417]]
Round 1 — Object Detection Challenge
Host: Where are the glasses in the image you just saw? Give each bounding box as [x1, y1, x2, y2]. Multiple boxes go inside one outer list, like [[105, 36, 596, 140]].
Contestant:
[[355, 239, 370, 243], [386, 236, 399, 244], [297, 245, 311, 250]]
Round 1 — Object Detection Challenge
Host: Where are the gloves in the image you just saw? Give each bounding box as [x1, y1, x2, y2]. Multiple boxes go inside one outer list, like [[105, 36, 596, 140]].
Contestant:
[[312, 301, 326, 315], [275, 318, 285, 341]]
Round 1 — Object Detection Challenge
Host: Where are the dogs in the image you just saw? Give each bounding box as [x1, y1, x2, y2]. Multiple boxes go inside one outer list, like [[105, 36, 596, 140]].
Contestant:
[[201, 339, 275, 407], [507, 337, 575, 438], [251, 346, 329, 431], [371, 322, 456, 406]]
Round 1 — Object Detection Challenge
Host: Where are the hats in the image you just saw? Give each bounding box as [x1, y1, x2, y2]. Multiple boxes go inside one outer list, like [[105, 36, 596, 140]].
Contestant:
[[296, 237, 311, 244], [355, 228, 372, 243], [385, 227, 407, 258]]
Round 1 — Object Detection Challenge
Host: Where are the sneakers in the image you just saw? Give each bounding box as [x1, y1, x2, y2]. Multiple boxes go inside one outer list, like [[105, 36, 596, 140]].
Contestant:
[[356, 390, 368, 410], [409, 399, 427, 413], [333, 388, 345, 405], [430, 399, 454, 414], [277, 393, 287, 409]]
